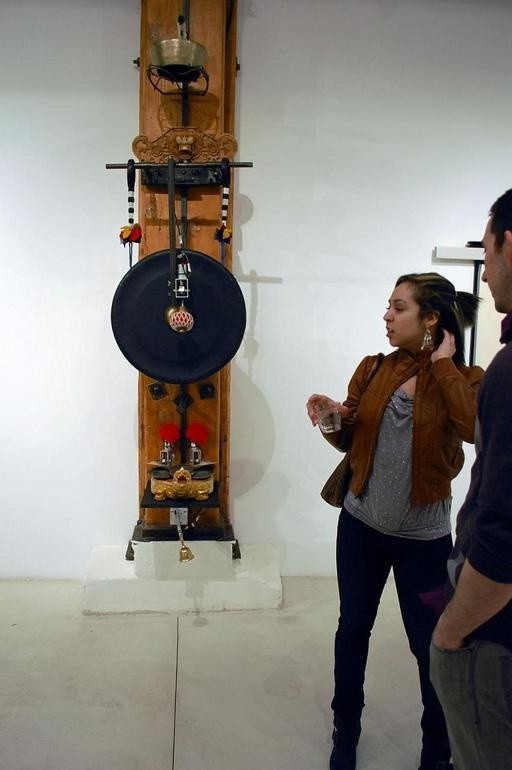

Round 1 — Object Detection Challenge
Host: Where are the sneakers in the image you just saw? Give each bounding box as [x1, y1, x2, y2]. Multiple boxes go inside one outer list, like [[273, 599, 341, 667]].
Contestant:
[[329, 729, 362, 770]]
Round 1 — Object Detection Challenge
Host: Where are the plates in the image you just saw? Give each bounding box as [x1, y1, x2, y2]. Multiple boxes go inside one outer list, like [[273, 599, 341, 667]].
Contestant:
[[148, 464, 216, 480]]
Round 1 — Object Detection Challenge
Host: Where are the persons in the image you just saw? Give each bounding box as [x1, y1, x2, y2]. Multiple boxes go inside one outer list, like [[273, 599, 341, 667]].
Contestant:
[[304, 272, 486, 770], [427, 187, 512, 769]]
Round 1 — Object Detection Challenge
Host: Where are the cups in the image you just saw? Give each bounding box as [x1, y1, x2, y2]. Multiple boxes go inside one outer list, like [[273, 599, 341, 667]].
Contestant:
[[315, 401, 342, 433]]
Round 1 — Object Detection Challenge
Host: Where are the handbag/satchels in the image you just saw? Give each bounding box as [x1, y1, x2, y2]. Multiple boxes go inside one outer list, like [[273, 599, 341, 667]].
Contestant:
[[320, 450, 352, 508]]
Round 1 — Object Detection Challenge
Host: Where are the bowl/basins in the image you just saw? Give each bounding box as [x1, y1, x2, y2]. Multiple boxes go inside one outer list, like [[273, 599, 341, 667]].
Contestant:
[[152, 35, 207, 83]]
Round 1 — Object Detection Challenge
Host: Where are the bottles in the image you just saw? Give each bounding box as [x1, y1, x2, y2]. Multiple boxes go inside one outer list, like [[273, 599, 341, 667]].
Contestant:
[[188, 442, 203, 462], [160, 441, 176, 464]]
